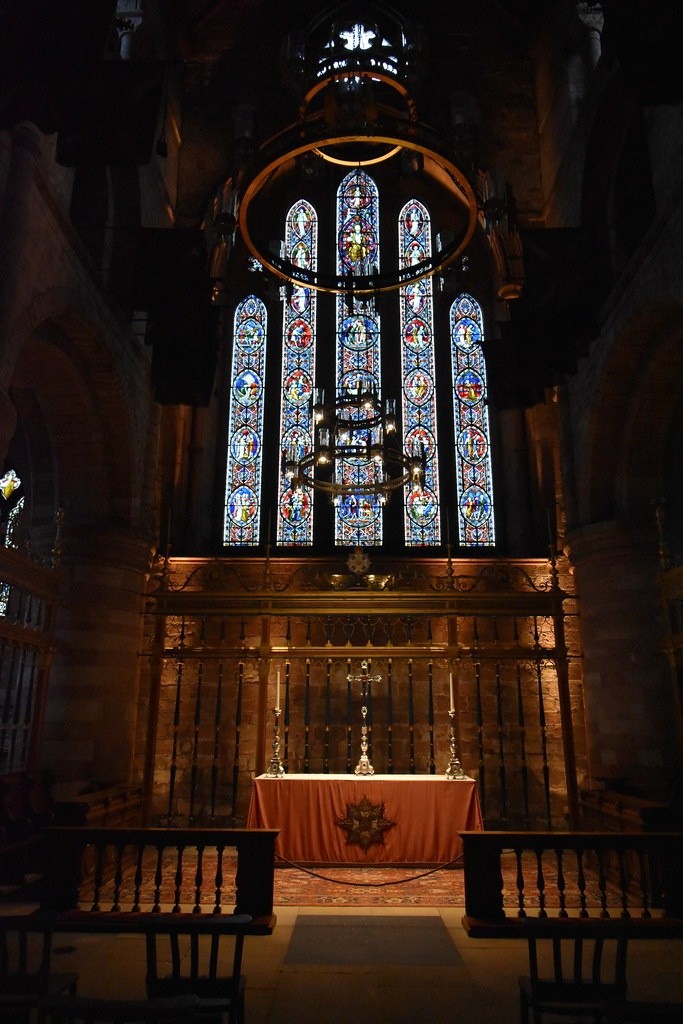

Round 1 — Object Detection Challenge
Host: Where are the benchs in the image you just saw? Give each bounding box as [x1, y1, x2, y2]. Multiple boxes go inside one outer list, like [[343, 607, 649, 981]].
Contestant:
[[0, 768, 71, 886]]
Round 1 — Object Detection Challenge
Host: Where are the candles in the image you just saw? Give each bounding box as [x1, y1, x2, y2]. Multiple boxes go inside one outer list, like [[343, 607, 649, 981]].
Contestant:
[[276, 671, 280, 711], [449, 672, 456, 714]]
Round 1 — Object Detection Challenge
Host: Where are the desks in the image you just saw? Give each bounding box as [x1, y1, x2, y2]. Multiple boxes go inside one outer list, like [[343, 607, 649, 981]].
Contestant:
[[246, 773, 484, 869]]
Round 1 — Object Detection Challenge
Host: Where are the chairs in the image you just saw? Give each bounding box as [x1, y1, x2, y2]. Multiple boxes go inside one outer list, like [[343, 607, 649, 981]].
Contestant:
[[138, 913, 257, 1024], [519, 918, 629, 1024], [0, 915, 82, 1024]]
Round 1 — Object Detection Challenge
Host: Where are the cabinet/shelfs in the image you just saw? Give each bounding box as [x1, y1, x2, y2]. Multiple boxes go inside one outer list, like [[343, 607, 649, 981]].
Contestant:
[[577, 789, 670, 903], [57, 784, 146, 897]]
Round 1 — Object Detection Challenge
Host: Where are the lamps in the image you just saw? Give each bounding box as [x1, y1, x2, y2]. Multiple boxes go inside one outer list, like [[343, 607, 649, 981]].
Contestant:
[[281, 378, 429, 511], [196, 0, 533, 314]]
